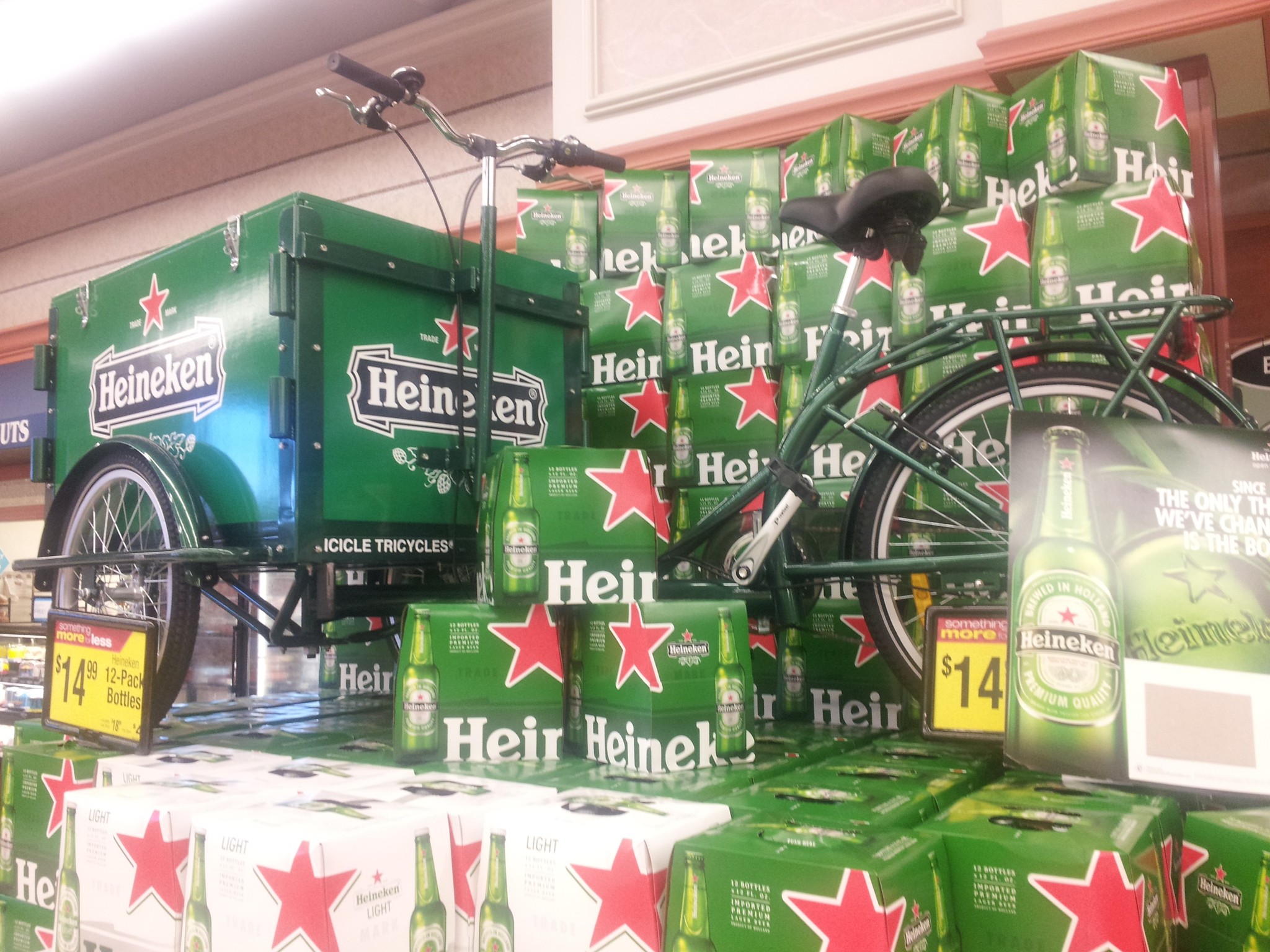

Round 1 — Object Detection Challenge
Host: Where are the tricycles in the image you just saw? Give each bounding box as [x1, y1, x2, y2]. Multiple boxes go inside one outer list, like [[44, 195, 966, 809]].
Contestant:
[[12, 53, 1260, 728]]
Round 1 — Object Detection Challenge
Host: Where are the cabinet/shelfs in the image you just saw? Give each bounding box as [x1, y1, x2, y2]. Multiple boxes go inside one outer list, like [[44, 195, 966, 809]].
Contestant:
[[0, 616, 235, 723]]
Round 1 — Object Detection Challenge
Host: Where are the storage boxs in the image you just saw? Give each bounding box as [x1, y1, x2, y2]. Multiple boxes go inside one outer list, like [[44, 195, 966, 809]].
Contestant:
[[0, 47, 1270, 952]]
